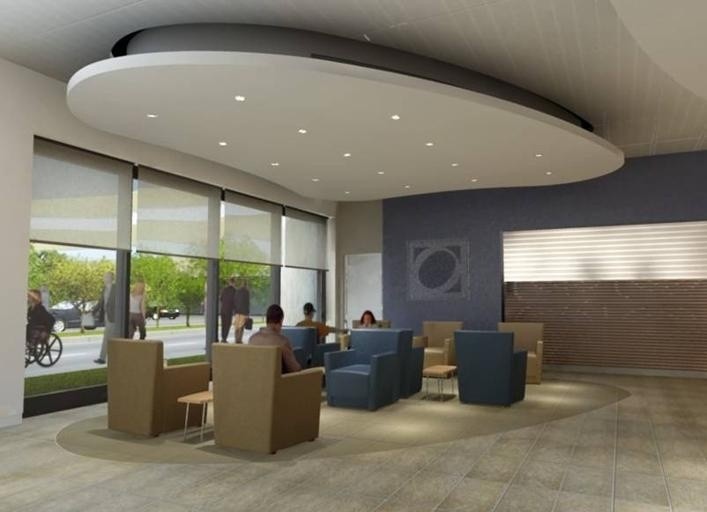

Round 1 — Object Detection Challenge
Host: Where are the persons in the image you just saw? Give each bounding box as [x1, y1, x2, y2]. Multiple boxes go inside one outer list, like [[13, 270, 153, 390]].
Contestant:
[[218, 278, 237, 344], [232, 278, 249, 343], [27, 289, 49, 343], [294, 302, 350, 345], [127, 281, 146, 340], [90, 271, 115, 364], [244, 304, 304, 373], [357, 310, 380, 330]]
